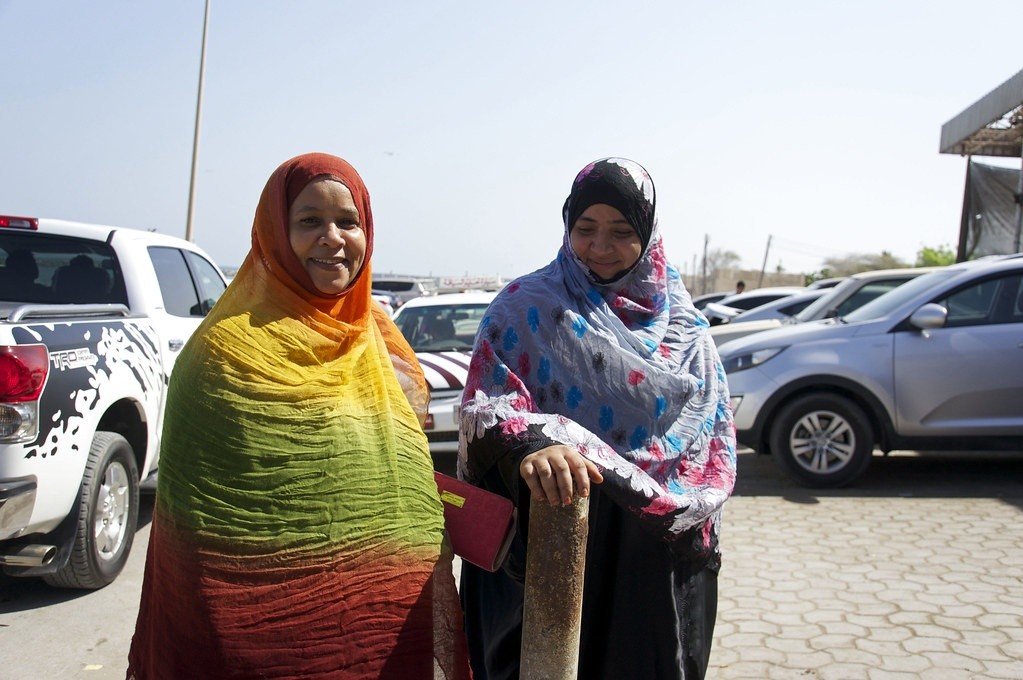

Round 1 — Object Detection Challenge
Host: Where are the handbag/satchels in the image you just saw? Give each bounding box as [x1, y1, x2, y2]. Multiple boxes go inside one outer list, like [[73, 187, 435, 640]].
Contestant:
[[434, 471, 521, 574]]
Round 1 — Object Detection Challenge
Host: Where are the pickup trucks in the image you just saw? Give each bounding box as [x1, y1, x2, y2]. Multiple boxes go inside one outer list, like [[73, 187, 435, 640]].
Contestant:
[[0, 210, 228, 591]]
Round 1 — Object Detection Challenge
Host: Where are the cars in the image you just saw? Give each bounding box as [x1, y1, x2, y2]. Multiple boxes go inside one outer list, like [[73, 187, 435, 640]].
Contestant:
[[370, 276, 483, 318], [390, 290, 499, 454], [690, 265, 986, 349]]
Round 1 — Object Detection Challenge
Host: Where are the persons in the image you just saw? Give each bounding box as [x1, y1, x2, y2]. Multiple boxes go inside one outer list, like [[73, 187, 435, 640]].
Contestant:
[[455, 159, 737, 680], [126, 152, 473, 680], [431, 320, 466, 346], [726, 281, 744, 297]]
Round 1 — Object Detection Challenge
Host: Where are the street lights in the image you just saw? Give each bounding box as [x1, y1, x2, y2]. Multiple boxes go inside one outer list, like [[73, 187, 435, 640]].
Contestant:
[[756, 233, 786, 288]]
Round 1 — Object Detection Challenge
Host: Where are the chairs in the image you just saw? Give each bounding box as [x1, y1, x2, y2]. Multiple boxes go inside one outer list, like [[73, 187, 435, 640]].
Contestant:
[[48, 267, 110, 303], [0, 268, 35, 302]]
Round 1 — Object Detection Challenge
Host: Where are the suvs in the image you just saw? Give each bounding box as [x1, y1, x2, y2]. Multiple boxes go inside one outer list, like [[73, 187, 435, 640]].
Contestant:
[[717, 252, 1023, 490]]
